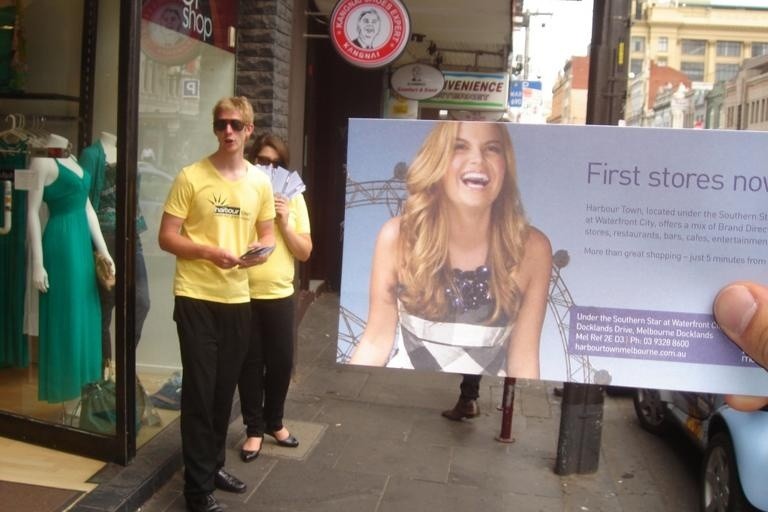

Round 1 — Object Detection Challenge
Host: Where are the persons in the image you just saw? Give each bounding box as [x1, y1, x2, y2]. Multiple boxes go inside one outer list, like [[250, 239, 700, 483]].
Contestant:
[[347, 120, 553, 381], [78, 131, 151, 381], [442, 375, 482, 420], [352, 10, 381, 50], [159, 96, 277, 512], [238, 134, 312, 461], [712, 281, 768, 412], [26, 132, 116, 404]]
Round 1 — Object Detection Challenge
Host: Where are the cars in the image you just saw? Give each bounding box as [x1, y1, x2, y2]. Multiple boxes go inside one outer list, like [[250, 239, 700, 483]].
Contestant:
[[134, 161, 179, 258], [632, 387, 768, 512]]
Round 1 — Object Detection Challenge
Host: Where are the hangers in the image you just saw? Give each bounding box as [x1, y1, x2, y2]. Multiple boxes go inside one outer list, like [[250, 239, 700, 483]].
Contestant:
[[0, 112, 73, 157]]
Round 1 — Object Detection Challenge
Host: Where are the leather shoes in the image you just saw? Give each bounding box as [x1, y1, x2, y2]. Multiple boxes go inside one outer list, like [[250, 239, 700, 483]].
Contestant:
[[240, 435, 264, 462], [214, 468, 247, 494], [266, 427, 299, 448], [442, 399, 480, 420], [185, 492, 223, 512]]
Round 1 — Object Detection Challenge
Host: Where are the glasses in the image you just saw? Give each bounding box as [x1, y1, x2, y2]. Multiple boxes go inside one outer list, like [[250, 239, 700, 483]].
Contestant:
[[256, 155, 283, 169], [215, 119, 245, 131]]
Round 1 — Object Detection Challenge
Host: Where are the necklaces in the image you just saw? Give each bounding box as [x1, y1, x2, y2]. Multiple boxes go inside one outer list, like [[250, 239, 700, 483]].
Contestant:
[[442, 264, 492, 311]]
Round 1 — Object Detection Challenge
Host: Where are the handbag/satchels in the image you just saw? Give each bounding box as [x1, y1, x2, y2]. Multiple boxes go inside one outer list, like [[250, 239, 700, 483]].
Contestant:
[[80, 374, 146, 439]]
